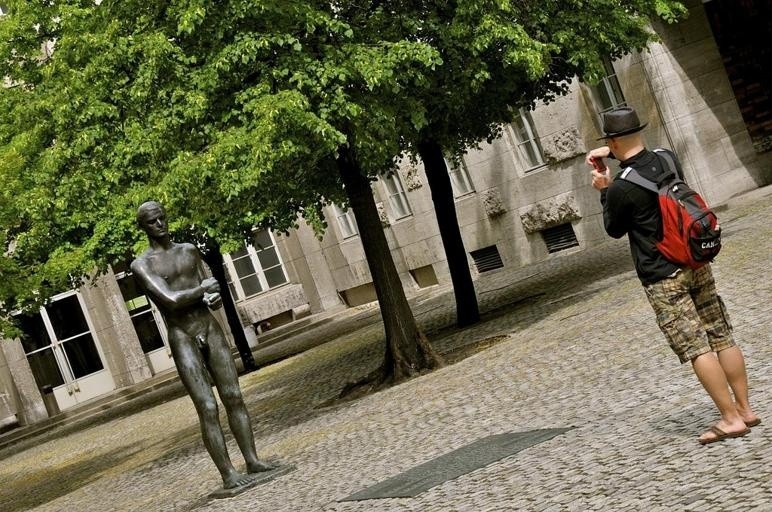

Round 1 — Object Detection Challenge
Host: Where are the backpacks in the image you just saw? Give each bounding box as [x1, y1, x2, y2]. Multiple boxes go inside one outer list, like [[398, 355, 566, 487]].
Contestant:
[[613, 148, 722, 271]]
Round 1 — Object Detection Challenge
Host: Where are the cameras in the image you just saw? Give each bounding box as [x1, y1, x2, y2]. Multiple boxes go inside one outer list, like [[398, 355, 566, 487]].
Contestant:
[[590, 156, 607, 173]]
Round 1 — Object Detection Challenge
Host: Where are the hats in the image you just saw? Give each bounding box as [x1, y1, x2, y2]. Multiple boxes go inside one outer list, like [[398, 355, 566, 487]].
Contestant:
[[595, 107, 649, 142]]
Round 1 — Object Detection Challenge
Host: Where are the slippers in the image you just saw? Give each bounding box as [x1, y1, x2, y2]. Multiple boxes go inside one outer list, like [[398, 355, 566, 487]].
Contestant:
[[697, 425, 751, 445], [744, 418, 761, 428]]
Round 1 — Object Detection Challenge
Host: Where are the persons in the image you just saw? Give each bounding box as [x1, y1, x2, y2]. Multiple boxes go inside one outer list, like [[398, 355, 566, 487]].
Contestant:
[[584, 107, 761, 446], [129, 200, 279, 490]]
